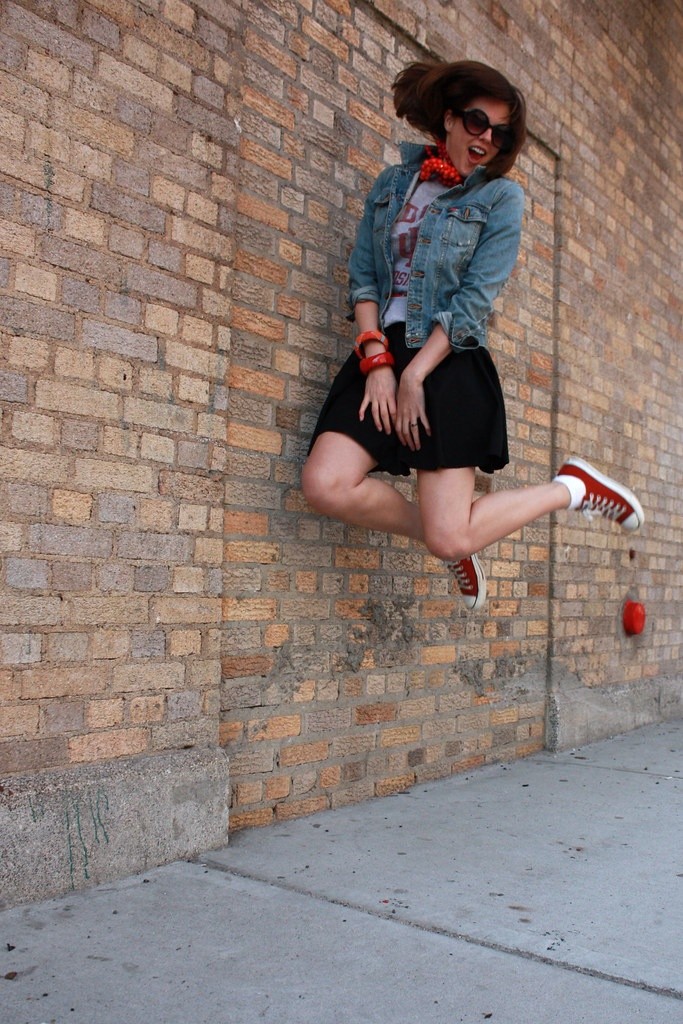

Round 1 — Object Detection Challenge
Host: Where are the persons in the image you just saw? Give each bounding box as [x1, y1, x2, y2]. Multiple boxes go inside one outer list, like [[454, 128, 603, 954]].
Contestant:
[[302, 57, 645, 612]]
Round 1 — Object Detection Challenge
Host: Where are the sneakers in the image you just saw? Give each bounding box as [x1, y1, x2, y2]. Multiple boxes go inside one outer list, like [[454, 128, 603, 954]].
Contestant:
[[445, 553, 486, 610], [557, 456, 645, 531]]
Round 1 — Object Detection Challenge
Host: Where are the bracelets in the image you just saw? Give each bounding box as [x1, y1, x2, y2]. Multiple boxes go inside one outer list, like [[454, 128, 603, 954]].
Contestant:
[[359, 351, 394, 377], [354, 330, 387, 359]]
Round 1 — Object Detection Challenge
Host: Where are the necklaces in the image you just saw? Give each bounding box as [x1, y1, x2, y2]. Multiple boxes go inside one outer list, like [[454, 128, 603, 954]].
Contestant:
[[419, 139, 461, 187]]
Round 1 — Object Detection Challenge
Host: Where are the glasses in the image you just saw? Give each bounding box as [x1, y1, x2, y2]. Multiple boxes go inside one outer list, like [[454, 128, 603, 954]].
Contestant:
[[450, 106, 509, 149]]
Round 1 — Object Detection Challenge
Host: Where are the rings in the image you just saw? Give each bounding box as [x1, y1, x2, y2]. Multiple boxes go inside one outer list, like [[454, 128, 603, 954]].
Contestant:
[[410, 423, 417, 428]]
